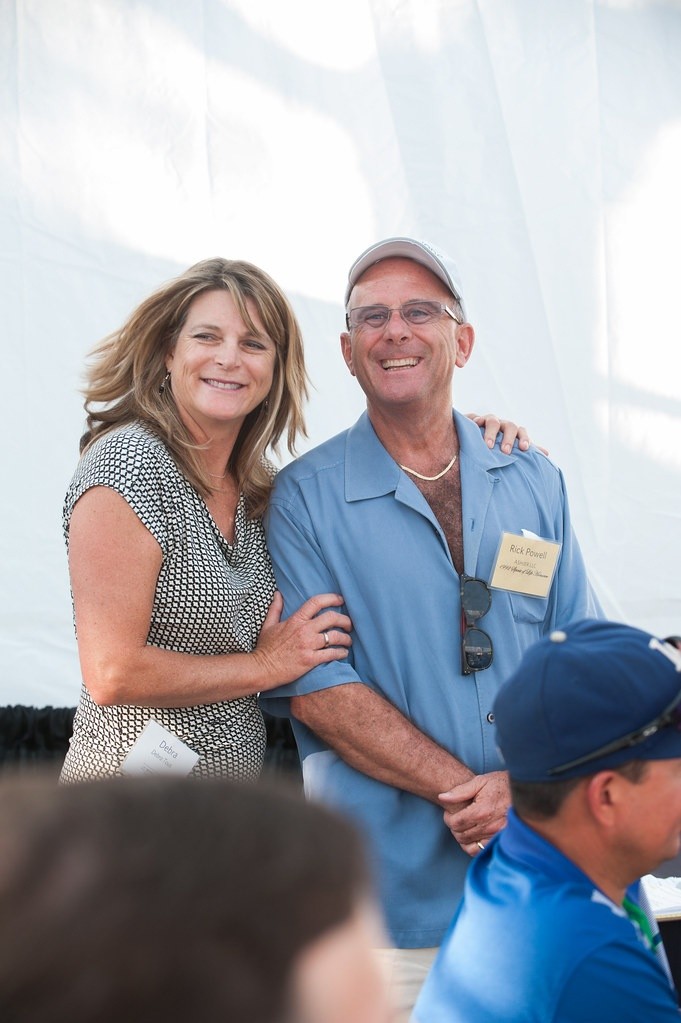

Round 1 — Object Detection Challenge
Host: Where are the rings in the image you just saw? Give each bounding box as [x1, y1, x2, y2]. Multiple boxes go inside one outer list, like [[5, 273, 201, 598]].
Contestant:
[[477, 842, 485, 849], [324, 633, 329, 648]]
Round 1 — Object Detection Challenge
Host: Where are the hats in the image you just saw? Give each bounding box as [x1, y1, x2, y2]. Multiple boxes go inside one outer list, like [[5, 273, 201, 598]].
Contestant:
[[492, 619, 681, 784], [348, 236, 464, 312]]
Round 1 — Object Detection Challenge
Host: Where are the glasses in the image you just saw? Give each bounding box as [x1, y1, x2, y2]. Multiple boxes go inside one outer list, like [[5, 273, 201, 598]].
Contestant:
[[458, 574, 494, 676], [345, 300, 461, 328]]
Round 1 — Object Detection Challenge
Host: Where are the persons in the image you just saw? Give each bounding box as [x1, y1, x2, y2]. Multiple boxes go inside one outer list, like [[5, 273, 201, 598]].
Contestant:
[[409, 619, 681, 1023], [0, 776, 398, 1023], [57, 235, 610, 1023]]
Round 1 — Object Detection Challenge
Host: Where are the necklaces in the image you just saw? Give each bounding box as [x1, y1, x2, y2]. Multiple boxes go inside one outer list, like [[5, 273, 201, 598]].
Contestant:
[[400, 455, 458, 480]]
